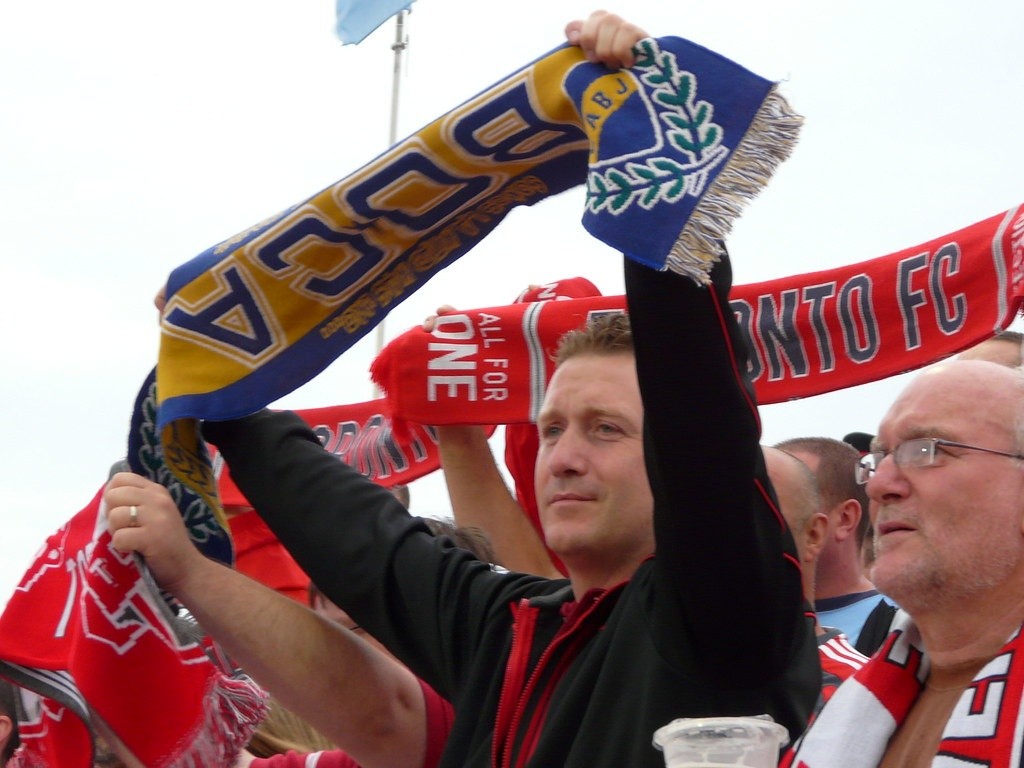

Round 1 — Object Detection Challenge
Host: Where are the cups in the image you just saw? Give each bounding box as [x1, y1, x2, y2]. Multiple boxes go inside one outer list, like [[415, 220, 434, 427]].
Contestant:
[[651, 715, 788, 768]]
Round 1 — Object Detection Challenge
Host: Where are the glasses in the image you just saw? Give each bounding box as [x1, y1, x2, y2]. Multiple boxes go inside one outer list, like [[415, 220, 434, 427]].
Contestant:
[[854, 437, 1024, 486]]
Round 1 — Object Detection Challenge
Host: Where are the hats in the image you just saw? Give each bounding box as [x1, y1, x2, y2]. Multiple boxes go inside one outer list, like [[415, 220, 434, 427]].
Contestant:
[[843, 432, 874, 457]]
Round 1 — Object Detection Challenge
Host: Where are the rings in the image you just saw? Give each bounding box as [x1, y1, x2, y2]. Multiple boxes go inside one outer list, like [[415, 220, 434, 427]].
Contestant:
[[128, 506, 136, 527]]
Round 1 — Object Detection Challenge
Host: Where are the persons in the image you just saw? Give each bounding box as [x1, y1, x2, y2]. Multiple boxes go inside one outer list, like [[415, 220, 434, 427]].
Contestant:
[[0, 9, 1024, 768]]
